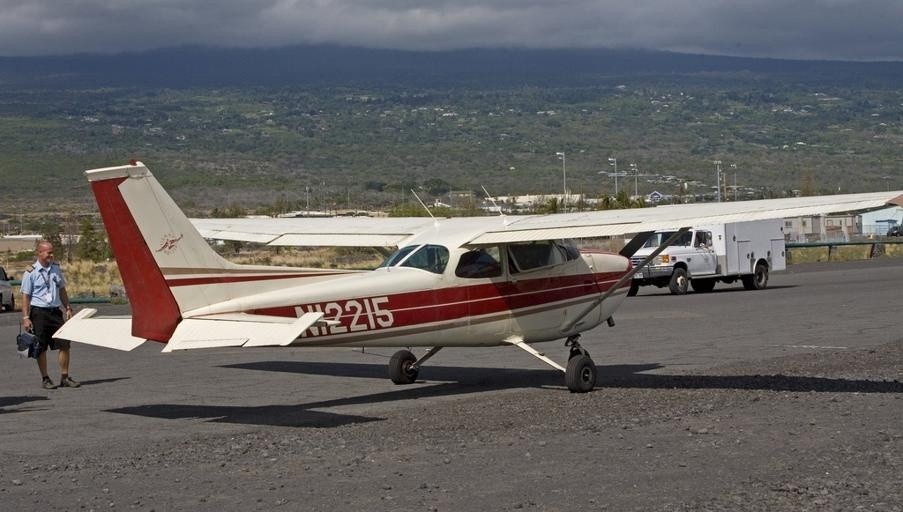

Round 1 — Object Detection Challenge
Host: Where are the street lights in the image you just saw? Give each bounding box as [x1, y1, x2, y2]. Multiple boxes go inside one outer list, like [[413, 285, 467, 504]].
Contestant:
[[550, 144, 742, 211]]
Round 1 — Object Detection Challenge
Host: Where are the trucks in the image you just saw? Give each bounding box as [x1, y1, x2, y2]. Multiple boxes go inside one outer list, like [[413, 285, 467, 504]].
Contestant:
[[620, 209, 788, 297]]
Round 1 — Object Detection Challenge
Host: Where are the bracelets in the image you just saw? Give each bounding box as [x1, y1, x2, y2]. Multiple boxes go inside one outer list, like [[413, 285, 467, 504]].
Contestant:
[[66, 308, 72, 312], [22, 316, 30, 320]]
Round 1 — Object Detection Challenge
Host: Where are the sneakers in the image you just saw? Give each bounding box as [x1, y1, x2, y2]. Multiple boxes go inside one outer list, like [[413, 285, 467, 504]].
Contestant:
[[41, 376, 57, 389], [59, 375, 83, 389]]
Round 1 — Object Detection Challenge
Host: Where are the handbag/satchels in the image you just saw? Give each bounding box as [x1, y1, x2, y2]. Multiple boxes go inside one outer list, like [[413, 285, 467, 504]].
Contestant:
[[15, 328, 38, 360]]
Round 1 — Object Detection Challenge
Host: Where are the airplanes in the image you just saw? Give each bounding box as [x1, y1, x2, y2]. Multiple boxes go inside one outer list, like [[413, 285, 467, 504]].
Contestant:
[[47, 151, 903, 392]]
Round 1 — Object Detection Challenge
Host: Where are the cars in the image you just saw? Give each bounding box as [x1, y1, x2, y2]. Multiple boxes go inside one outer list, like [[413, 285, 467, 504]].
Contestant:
[[884, 224, 902, 238], [0, 266, 14, 312]]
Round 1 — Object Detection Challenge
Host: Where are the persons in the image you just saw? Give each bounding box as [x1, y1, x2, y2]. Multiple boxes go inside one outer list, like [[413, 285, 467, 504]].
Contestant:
[[19, 239, 81, 389], [688, 234, 701, 247]]
